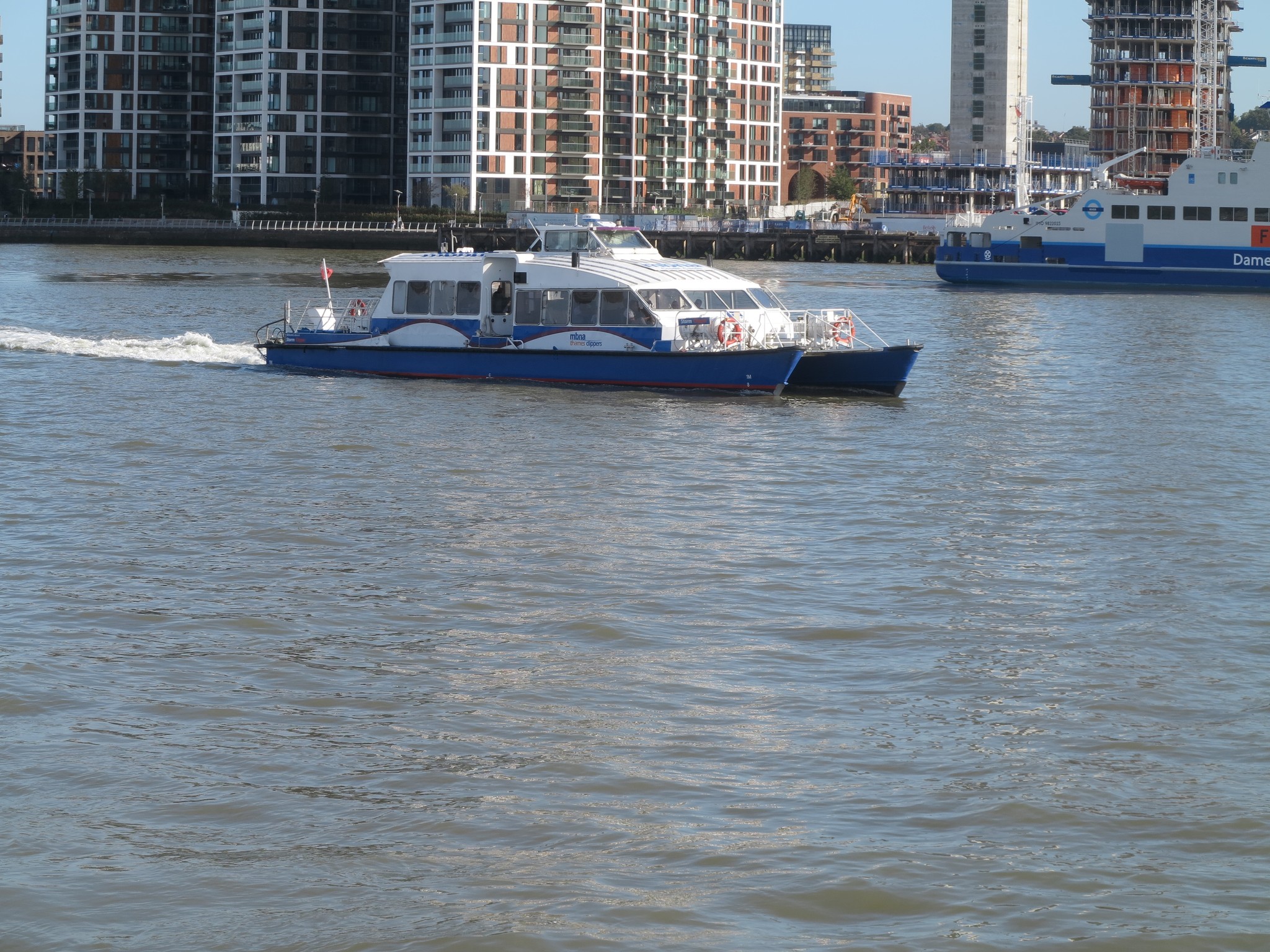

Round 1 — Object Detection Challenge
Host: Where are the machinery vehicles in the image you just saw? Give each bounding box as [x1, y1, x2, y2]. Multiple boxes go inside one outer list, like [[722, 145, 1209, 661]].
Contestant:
[[785, 193, 873, 235]]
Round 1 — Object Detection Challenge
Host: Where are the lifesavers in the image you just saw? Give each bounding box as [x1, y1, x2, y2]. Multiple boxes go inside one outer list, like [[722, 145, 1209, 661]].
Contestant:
[[351, 299, 366, 316], [717, 317, 741, 347], [832, 317, 855, 344]]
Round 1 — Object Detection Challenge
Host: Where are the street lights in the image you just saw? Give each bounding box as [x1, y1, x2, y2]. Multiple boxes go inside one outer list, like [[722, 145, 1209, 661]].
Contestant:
[[86, 189, 95, 224], [890, 147, 897, 168], [453, 193, 458, 227], [232, 188, 241, 227], [475, 191, 484, 227], [461, 180, 465, 212], [311, 189, 320, 228], [393, 190, 403, 230], [564, 192, 572, 213], [798, 159, 804, 211], [761, 192, 770, 220], [825, 162, 828, 211], [650, 192, 660, 214], [160, 194, 165, 226], [18, 188, 27, 218]]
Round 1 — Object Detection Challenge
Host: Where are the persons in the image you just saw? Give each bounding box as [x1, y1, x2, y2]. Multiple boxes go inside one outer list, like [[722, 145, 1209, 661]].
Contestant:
[[572, 296, 657, 326], [693, 298, 703, 310], [459, 286, 480, 314], [671, 301, 679, 309], [492, 284, 511, 317]]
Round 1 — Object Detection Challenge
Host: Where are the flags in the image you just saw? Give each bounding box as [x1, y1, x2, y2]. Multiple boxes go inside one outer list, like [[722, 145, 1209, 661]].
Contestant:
[[321, 261, 333, 279]]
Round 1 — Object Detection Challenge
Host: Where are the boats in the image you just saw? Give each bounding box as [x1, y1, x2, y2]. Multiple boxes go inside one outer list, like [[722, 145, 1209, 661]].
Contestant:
[[1113, 173, 1169, 190], [931, 138, 1270, 292], [253, 207, 926, 397]]
